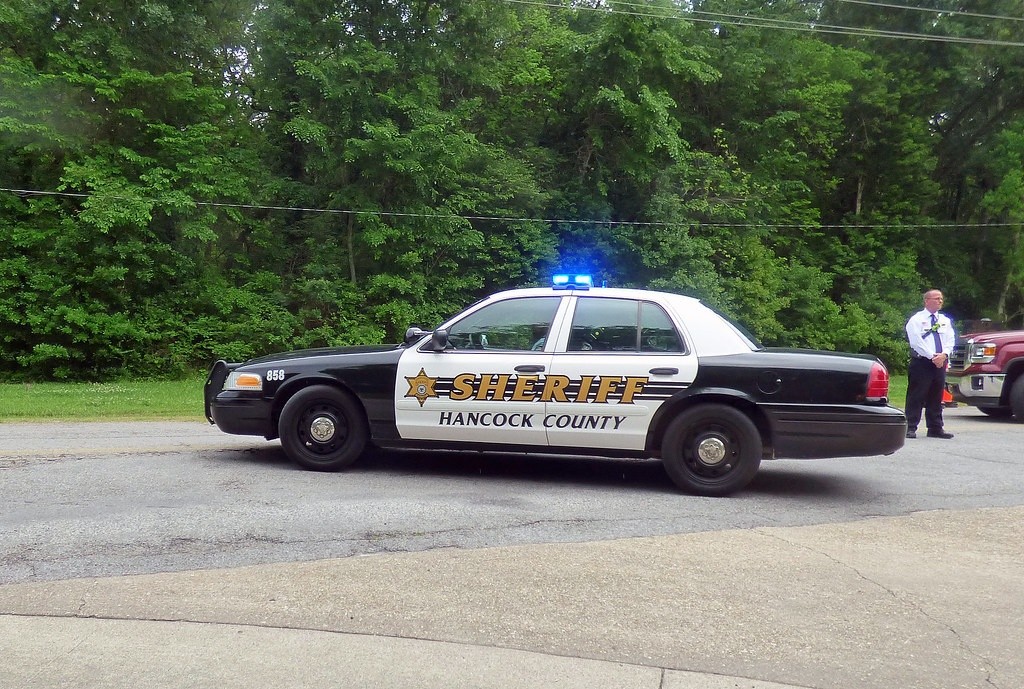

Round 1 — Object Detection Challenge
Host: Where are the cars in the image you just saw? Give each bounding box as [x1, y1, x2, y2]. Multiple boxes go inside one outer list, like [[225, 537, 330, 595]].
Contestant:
[[205, 275, 908, 497]]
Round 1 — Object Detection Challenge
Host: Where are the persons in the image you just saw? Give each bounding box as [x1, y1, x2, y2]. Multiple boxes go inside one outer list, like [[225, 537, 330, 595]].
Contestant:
[[905, 290, 955, 439], [531, 318, 548, 352]]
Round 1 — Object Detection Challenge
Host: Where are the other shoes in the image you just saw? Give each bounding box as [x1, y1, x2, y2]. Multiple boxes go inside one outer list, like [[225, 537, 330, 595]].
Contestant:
[[927, 428, 953, 438], [905, 427, 915, 438]]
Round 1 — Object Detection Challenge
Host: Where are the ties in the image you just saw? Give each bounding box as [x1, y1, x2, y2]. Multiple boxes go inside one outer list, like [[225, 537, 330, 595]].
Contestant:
[[930, 314, 943, 353]]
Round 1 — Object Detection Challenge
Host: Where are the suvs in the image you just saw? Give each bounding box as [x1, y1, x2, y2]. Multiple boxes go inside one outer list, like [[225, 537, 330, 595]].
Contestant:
[[944, 330, 1024, 423]]
